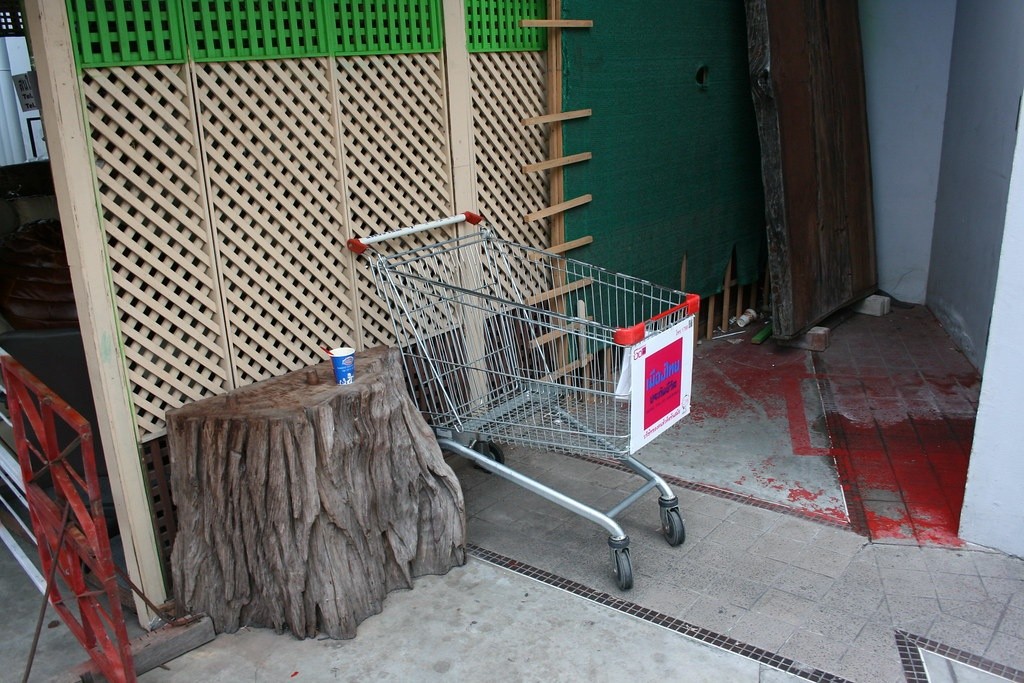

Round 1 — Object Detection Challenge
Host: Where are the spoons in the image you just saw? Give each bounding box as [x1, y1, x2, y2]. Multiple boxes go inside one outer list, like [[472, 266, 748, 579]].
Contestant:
[[321, 345, 334, 355]]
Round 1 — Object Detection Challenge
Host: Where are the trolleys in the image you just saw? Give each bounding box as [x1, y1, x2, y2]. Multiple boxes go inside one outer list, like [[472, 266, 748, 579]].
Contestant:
[[344, 211, 699, 592]]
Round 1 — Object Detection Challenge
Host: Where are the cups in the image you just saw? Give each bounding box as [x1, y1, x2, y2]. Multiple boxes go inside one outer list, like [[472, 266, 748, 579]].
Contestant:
[[329, 347, 356, 385], [736, 307, 758, 327]]
